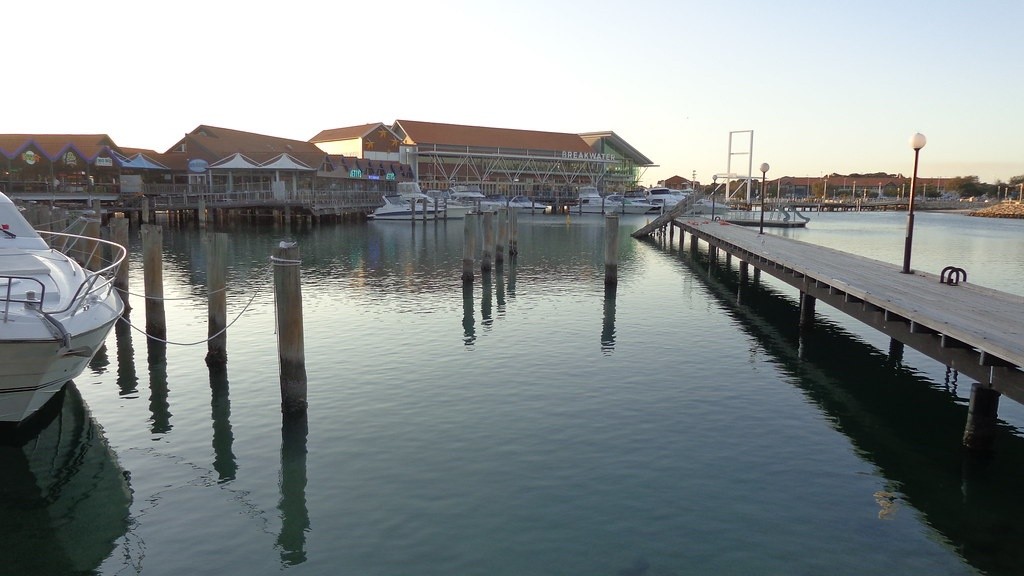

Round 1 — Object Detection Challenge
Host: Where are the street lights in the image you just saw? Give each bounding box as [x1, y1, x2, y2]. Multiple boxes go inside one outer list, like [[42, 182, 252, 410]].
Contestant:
[[712, 175, 718, 220], [898, 132, 926, 275], [691, 170, 696, 218], [759, 162, 770, 235]]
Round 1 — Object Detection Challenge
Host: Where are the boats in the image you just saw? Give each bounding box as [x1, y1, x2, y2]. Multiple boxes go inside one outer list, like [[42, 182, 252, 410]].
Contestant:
[[0, 191, 126, 425], [367, 173, 735, 228], [0, 381, 137, 576]]
